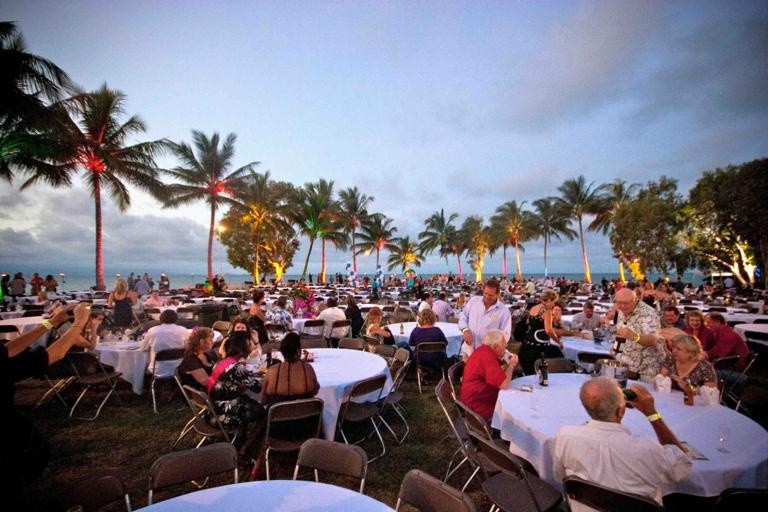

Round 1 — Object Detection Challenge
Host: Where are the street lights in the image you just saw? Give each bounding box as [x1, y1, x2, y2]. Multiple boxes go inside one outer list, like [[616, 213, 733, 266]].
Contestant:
[[216, 224, 224, 274], [116, 273, 120, 278], [59, 272, 65, 294], [190, 271, 195, 289]]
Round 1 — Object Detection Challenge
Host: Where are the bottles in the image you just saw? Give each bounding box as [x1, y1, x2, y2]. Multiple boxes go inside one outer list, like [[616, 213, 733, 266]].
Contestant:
[[621, 388, 638, 403], [611, 322, 627, 352], [16, 302, 21, 313], [536, 351, 549, 386], [399, 323, 404, 334], [591, 320, 610, 344], [699, 380, 720, 406], [681, 379, 694, 406]]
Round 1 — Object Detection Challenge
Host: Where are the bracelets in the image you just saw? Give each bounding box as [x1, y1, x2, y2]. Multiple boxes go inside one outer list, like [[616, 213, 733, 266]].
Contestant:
[[647, 413, 662, 422]]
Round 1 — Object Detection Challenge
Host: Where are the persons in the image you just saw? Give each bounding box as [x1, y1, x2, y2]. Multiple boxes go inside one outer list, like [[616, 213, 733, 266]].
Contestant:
[[600, 275, 766, 395], [554, 375, 693, 511], [209, 274, 348, 479], [333, 270, 602, 430], [179, 326, 219, 404], [109, 274, 188, 404], [2, 274, 106, 511]]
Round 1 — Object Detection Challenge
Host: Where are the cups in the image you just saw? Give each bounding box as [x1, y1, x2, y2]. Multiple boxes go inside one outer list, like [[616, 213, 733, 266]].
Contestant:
[[94, 328, 146, 345], [594, 358, 630, 391], [271, 348, 280, 359]]
